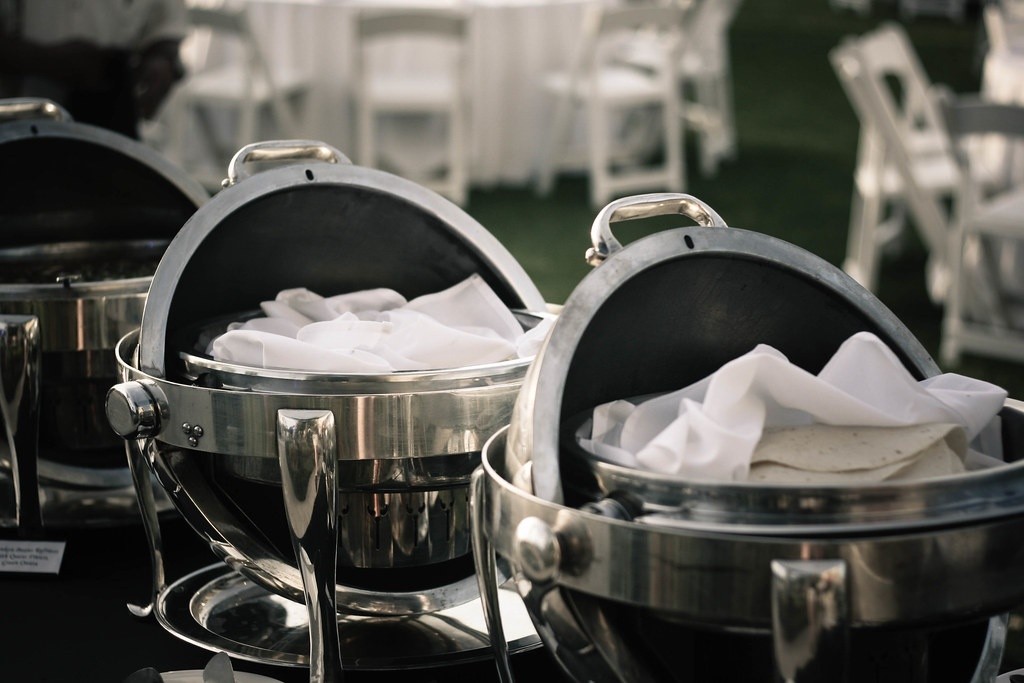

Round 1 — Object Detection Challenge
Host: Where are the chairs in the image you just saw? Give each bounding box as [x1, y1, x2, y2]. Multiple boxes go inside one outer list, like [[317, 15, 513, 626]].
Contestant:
[[832, 0, 1024, 364], [541, 0, 746, 204], [347, 0, 467, 208], [175, 0, 313, 183]]
